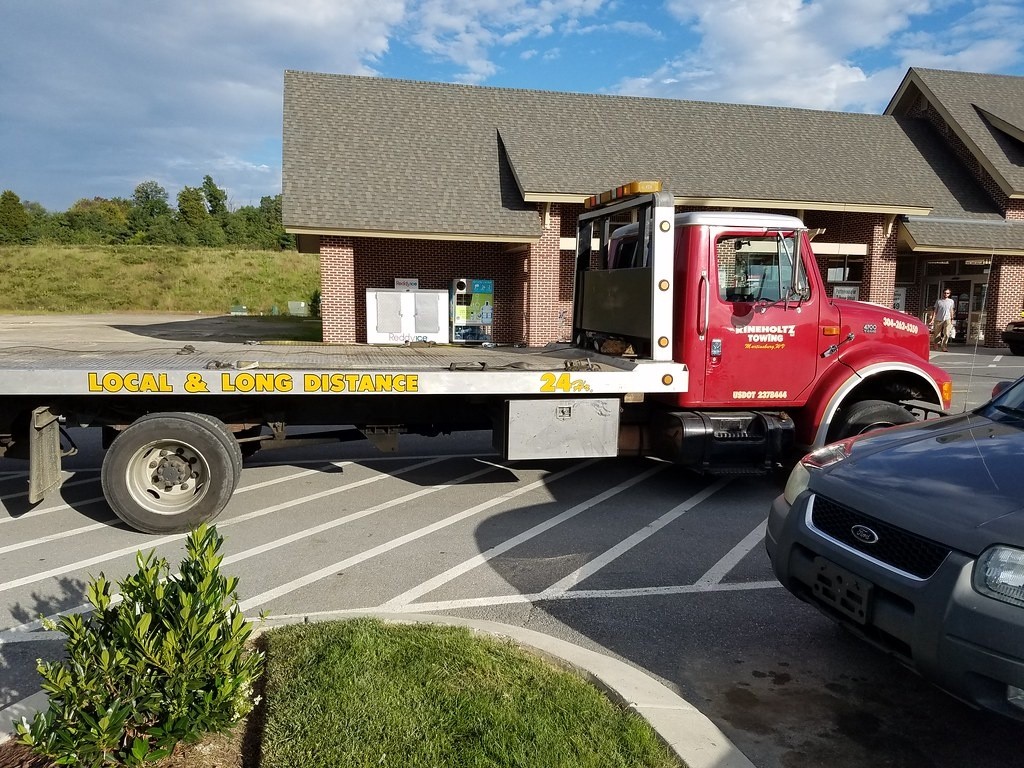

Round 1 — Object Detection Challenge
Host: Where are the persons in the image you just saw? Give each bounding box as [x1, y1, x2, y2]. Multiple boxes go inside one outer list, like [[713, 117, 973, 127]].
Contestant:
[[929, 288, 954, 352]]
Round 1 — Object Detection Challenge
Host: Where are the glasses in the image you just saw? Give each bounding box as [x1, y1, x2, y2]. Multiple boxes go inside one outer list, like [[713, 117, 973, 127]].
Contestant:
[[944, 292, 950, 294]]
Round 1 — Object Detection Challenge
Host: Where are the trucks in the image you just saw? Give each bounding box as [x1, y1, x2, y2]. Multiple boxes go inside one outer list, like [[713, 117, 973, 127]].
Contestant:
[[1, 180, 954, 537]]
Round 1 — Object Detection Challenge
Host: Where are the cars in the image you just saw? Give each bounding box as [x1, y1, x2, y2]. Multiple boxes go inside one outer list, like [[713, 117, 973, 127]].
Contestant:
[[1001, 320, 1024, 356], [764, 375, 1024, 731]]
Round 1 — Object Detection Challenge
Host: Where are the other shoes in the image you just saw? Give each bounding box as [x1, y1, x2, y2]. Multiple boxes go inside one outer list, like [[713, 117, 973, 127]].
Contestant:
[[942, 347, 949, 352], [933, 344, 939, 350]]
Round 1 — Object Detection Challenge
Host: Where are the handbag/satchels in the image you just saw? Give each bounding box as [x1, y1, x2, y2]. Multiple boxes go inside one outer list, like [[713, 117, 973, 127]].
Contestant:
[[951, 327, 955, 338]]
